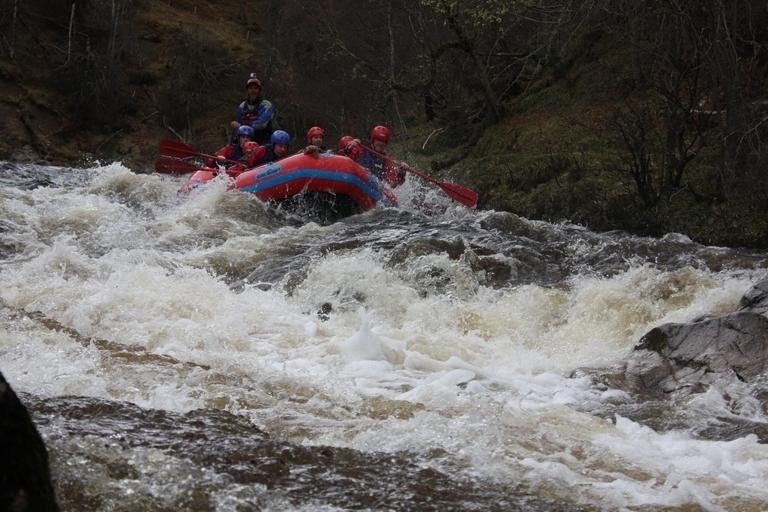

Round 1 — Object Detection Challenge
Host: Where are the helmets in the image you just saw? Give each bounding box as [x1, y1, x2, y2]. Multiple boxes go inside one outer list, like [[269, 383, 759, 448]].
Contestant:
[[270, 130, 289, 148], [338, 136, 353, 148], [307, 127, 323, 140], [243, 143, 258, 153], [239, 126, 254, 138], [371, 126, 389, 143], [245, 72, 264, 89]]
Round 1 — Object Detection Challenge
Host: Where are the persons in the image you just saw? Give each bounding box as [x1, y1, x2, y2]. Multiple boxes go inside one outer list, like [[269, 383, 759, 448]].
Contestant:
[[207, 125, 410, 188], [231, 79, 274, 146]]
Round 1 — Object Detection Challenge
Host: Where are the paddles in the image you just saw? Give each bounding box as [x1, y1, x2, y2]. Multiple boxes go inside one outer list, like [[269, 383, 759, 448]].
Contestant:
[[159, 140, 239, 164], [356, 142, 476, 207], [155, 159, 245, 174]]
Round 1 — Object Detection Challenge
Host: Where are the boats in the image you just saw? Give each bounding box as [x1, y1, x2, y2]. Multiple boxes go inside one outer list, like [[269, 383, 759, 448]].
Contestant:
[[176, 144, 399, 221]]
